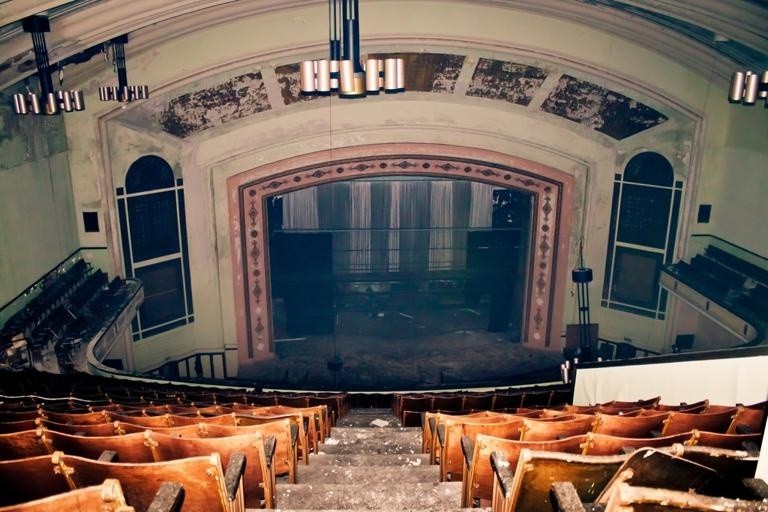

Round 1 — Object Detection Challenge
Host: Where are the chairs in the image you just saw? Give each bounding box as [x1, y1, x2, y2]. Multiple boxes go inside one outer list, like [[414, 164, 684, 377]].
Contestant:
[[0, 258, 352, 512], [391, 385, 768, 512]]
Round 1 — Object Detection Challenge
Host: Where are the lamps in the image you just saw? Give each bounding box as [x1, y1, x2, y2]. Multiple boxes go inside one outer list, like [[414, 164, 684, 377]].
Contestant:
[[12, 15, 85, 116], [727, 70, 768, 109], [98, 31, 149, 102], [298, 0, 406, 99]]
